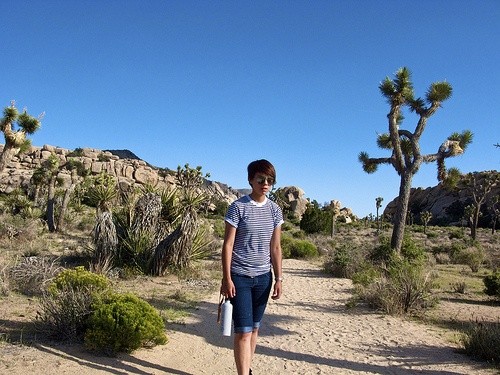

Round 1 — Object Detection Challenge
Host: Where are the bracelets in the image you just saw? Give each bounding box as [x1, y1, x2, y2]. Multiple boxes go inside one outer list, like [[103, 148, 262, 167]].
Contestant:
[[274, 276, 282, 282]]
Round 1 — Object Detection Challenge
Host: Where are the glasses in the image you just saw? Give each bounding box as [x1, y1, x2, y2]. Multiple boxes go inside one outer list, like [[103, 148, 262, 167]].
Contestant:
[[253, 176, 274, 185]]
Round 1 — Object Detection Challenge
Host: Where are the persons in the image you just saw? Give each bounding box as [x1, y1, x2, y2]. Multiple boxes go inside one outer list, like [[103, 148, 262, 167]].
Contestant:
[[221, 159, 284, 375]]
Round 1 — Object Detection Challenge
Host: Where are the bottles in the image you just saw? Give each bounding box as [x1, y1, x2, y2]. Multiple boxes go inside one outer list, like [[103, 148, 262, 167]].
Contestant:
[[220, 298, 233, 336]]
